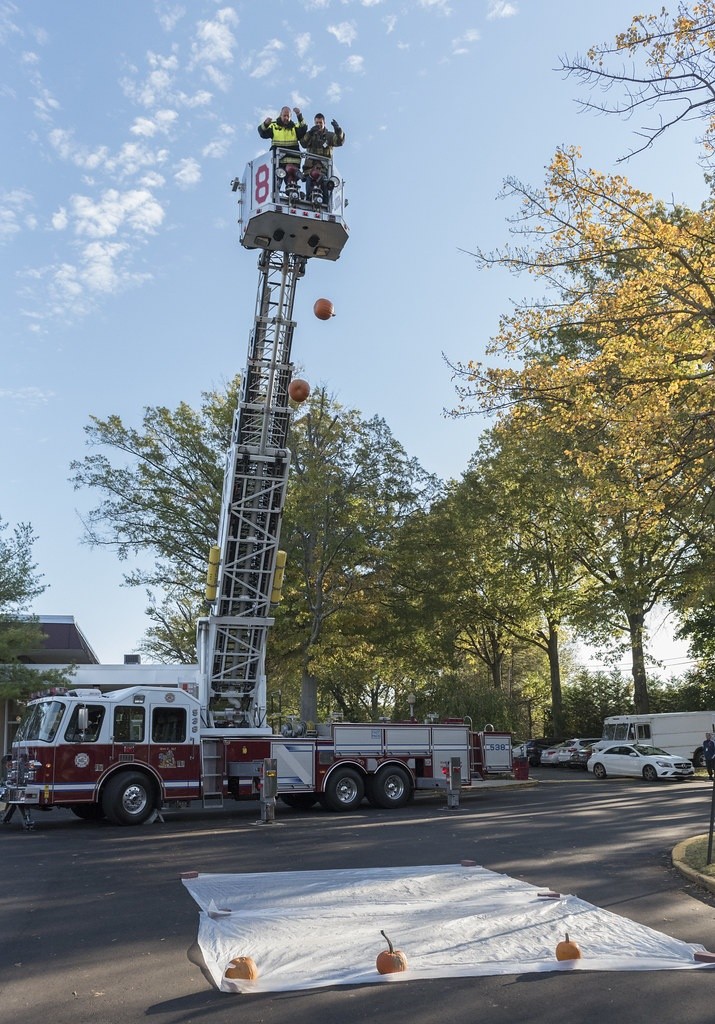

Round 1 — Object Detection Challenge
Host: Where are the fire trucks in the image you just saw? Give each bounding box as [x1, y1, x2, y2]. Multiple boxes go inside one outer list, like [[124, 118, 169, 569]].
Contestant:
[[0, 145, 483, 826]]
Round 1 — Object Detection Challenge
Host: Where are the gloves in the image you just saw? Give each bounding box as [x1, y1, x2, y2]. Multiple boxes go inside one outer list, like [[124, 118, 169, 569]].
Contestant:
[[309, 125, 320, 136], [331, 119, 340, 131]]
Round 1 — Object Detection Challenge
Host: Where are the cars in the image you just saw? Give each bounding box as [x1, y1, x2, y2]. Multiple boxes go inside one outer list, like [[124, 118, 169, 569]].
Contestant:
[[587, 743, 695, 780], [511, 736, 601, 770]]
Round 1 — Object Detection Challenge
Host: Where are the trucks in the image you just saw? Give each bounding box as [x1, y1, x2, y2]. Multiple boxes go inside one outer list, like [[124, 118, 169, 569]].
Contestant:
[[591, 711, 715, 767]]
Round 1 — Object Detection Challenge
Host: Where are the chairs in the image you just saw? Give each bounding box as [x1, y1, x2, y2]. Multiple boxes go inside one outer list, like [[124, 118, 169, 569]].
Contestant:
[[155, 716, 179, 743], [80, 712, 98, 742]]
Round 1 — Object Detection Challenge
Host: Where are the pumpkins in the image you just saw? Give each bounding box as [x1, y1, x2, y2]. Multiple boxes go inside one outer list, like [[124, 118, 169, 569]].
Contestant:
[[288, 380, 310, 402], [314, 298, 336, 320], [226, 957, 257, 981], [376, 930, 407, 975], [556, 933, 580, 962]]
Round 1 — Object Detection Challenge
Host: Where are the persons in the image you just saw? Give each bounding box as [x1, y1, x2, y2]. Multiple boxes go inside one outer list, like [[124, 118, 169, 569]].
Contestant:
[[702, 732, 715, 780], [2, 755, 14, 785]]
